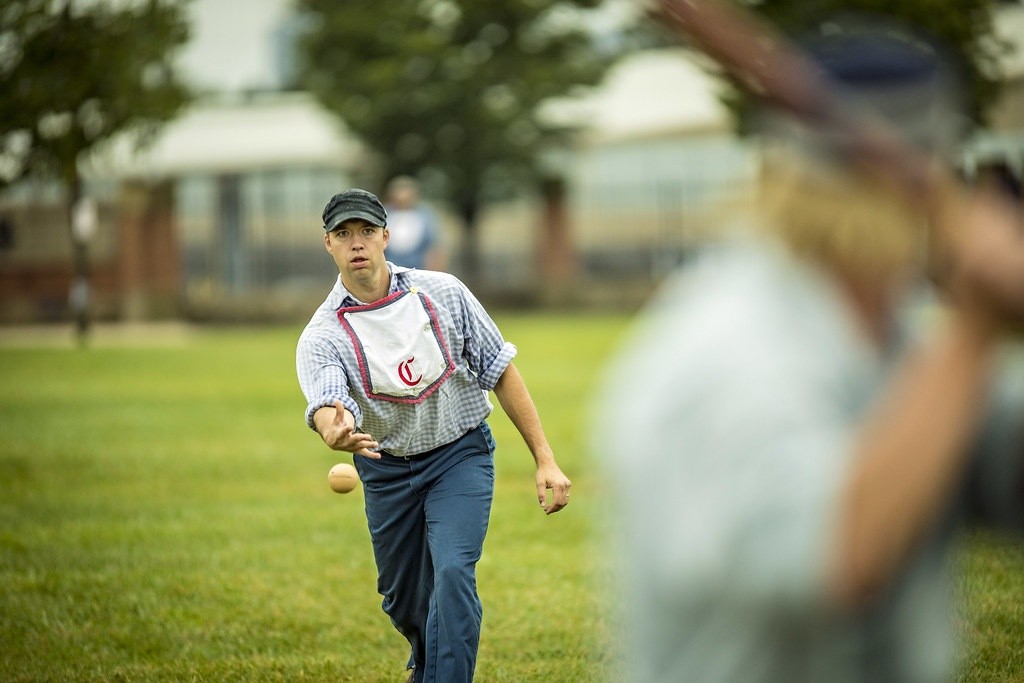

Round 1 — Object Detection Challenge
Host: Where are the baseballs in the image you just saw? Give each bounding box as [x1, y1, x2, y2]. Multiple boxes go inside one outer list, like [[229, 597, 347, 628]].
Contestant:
[[329, 462, 359, 494]]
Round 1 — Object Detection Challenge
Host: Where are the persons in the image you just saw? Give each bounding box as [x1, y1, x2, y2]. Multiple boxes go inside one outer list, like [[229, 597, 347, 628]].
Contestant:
[[296, 188, 572, 683], [594, 28, 1024, 683], [381, 174, 438, 267]]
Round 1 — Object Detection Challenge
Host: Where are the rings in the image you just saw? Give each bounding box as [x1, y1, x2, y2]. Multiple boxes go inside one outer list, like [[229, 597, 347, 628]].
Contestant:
[[566, 494, 571, 497]]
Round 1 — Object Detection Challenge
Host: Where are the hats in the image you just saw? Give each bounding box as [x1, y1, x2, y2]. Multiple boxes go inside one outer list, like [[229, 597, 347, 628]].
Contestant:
[[321, 186, 388, 233]]
